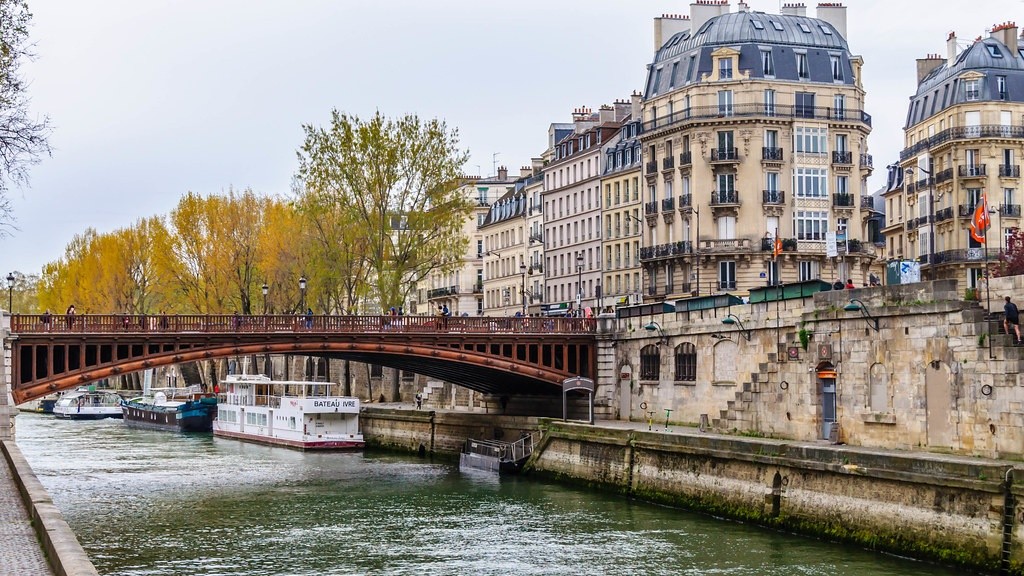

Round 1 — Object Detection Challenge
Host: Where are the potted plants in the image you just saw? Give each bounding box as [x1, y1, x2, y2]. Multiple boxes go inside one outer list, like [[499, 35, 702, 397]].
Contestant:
[[783, 238, 797, 251]]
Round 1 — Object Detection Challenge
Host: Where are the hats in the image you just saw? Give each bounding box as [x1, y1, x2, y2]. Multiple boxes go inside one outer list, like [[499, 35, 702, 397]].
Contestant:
[[390, 307, 395, 310]]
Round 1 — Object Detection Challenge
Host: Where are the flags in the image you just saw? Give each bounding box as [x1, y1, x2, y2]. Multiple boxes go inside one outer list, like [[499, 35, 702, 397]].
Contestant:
[[970, 191, 992, 245], [773, 235, 783, 261]]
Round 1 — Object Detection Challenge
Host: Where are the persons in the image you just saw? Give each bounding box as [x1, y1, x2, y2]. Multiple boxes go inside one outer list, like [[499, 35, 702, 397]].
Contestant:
[[1003, 296, 1022, 344], [41, 308, 52, 330], [524, 313, 532, 331], [161, 311, 168, 329], [306, 308, 314, 328], [390, 307, 397, 326], [398, 306, 404, 320], [122, 312, 130, 330], [416, 390, 423, 410], [431, 306, 449, 331], [290, 309, 297, 325], [65, 305, 76, 329], [233, 310, 241, 330], [384, 313, 391, 329], [442, 304, 448, 330], [846, 278, 857, 290], [565, 309, 580, 331]]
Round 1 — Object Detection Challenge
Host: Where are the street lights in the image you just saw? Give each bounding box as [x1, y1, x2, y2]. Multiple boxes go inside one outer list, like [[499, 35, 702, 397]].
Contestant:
[[685, 204, 699, 297], [519, 261, 527, 316], [7, 274, 15, 314], [905, 164, 937, 281], [988, 201, 1003, 276], [576, 254, 584, 317], [299, 275, 306, 315], [626, 210, 645, 298], [261, 284, 269, 314]]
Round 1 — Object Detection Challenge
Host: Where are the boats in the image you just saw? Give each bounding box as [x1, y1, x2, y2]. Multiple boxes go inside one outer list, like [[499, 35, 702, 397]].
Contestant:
[[53, 386, 129, 420], [212, 356, 366, 451], [15, 388, 74, 413], [120, 386, 218, 432]]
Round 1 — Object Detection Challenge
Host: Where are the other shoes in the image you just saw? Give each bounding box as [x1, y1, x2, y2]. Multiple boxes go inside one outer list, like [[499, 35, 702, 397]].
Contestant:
[[1017, 340, 1022, 345]]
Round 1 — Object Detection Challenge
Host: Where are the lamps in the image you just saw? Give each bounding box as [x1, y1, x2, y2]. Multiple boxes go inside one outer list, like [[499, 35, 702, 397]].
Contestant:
[[529, 237, 543, 243], [645, 321, 668, 346], [843, 299, 878, 332], [486, 251, 500, 258], [626, 215, 641, 222], [721, 314, 750, 341]]
